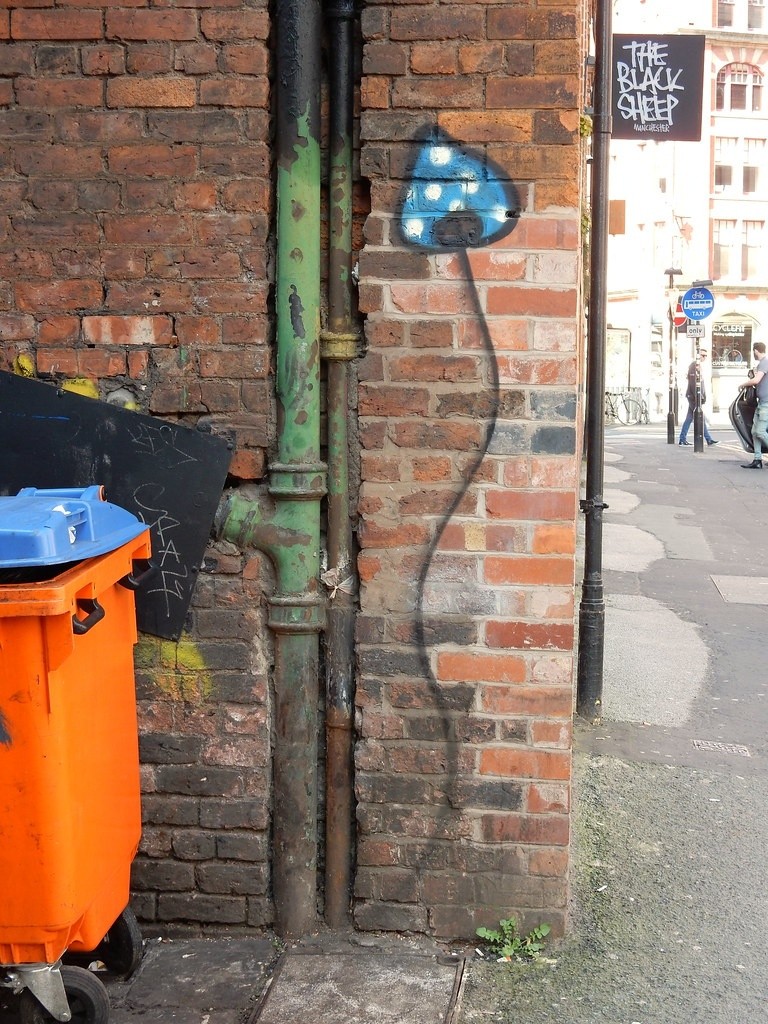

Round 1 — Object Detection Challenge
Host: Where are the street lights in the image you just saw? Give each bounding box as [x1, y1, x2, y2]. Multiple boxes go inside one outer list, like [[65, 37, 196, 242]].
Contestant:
[[663, 268, 683, 445]]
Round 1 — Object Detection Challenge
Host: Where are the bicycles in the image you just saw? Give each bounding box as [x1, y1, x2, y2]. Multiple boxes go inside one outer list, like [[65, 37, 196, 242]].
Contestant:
[[605, 388, 651, 426]]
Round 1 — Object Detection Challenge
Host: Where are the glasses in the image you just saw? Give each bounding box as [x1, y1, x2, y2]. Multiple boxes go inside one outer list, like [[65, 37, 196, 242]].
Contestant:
[[701, 354, 707, 357]]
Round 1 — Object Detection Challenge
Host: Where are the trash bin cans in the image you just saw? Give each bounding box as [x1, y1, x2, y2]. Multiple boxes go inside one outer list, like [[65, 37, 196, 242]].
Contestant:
[[0, 483, 153, 1024]]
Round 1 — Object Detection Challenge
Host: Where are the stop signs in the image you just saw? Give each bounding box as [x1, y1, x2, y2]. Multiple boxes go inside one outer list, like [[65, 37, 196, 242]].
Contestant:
[[667, 302, 688, 327]]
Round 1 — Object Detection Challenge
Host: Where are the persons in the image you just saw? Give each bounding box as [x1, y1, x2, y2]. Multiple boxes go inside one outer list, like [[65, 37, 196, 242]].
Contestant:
[[679, 349, 719, 445], [738, 342, 768, 468], [733, 351, 740, 361]]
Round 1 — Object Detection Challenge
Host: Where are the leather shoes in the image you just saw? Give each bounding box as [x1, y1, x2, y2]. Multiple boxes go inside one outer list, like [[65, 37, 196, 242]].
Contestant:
[[741, 460, 762, 469], [679, 441, 693, 446], [765, 462, 768, 466], [707, 440, 718, 445]]
